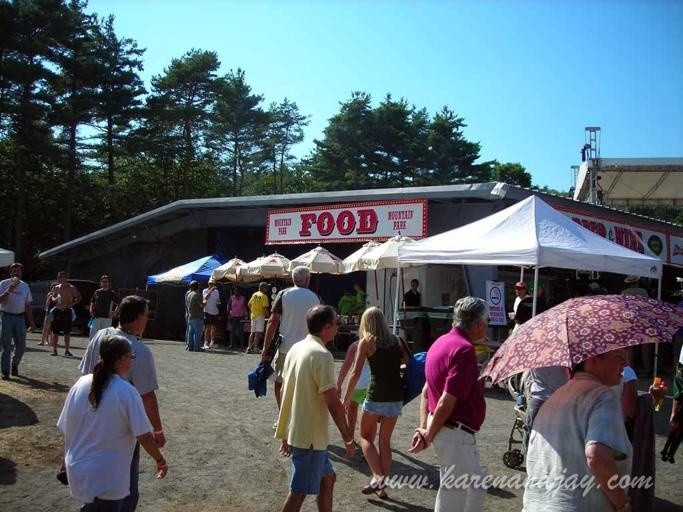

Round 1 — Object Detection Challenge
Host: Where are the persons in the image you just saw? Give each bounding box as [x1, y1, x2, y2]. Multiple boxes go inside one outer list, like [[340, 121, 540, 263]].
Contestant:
[[521, 347, 632, 512], [52, 336, 166, 510], [262, 268, 320, 430], [506, 281, 683, 463], [184, 279, 268, 354], [343, 307, 409, 499], [337, 337, 370, 445], [403, 278, 420, 307], [272, 304, 357, 511], [407, 298, 492, 512], [73, 294, 165, 511], [0, 263, 122, 380]]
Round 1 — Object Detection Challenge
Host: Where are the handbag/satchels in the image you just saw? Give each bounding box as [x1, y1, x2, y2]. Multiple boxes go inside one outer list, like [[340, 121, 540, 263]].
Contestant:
[[400, 351, 427, 407]]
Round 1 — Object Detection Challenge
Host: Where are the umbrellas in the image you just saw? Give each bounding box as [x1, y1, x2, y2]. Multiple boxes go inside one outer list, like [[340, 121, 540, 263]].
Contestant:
[[360, 231, 428, 336], [238, 254, 270, 283], [208, 257, 245, 288], [476, 294, 681, 382], [287, 243, 342, 296], [338, 237, 382, 307], [143, 253, 230, 288], [247, 251, 292, 288]]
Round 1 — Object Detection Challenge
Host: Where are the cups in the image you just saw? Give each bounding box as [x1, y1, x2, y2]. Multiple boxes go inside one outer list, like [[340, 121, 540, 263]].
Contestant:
[[245, 316, 248, 320], [652, 387, 670, 413], [10, 278, 16, 292]]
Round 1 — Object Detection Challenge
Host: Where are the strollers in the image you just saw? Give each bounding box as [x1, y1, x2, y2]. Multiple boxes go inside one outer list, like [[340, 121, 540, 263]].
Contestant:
[[503, 372, 533, 470]]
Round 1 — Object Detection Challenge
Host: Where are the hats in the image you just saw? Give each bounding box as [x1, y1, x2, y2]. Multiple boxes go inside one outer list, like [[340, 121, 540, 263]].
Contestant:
[[208, 279, 216, 284], [259, 282, 272, 288], [623, 275, 641, 284], [514, 281, 528, 288]]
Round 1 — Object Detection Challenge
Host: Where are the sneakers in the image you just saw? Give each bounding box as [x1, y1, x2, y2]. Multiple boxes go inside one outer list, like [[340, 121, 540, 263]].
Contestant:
[[186, 341, 260, 355], [37, 342, 46, 346], [50, 350, 58, 356], [1, 371, 10, 380], [11, 363, 18, 376], [64, 350, 72, 356]]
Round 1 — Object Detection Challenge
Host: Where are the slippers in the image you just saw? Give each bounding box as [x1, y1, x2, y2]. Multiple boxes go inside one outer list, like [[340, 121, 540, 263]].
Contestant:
[[373, 489, 388, 499], [362, 479, 388, 495]]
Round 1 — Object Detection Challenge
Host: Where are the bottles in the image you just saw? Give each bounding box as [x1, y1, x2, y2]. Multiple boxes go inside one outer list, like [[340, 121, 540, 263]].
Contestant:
[[212, 286, 217, 292]]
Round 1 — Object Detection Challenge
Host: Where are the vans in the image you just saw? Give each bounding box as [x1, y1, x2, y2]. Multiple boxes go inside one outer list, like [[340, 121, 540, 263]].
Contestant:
[[25, 278, 99, 338]]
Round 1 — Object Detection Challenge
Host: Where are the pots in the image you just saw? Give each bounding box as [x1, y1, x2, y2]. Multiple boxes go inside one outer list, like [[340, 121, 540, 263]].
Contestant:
[[337, 314, 359, 324]]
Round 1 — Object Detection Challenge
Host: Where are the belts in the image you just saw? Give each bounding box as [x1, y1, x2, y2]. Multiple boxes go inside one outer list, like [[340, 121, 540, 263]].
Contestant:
[[447, 421, 475, 435]]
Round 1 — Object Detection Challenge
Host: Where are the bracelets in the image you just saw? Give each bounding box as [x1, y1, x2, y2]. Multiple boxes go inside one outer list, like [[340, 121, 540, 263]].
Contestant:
[[342, 437, 352, 447], [613, 495, 630, 510], [154, 461, 166, 468], [153, 427, 164, 435]]
